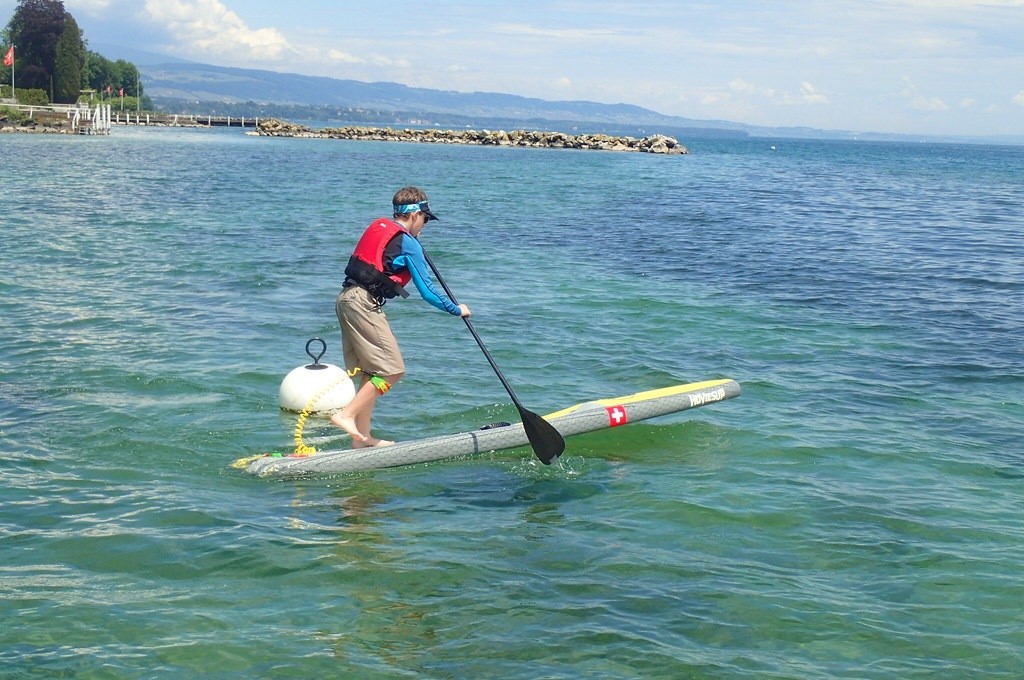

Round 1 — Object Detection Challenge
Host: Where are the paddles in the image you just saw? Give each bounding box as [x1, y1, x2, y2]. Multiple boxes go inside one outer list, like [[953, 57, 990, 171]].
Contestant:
[[414, 235, 565, 465]]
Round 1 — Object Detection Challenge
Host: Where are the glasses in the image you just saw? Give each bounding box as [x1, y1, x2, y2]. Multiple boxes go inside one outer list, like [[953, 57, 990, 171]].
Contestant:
[[421, 212, 430, 223]]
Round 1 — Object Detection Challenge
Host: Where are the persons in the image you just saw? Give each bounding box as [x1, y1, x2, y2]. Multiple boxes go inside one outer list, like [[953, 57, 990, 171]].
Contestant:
[[330, 187, 471, 449]]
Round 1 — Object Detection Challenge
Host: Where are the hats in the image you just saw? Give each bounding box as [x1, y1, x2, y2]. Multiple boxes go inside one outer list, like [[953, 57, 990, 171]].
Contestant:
[[393, 200, 440, 221]]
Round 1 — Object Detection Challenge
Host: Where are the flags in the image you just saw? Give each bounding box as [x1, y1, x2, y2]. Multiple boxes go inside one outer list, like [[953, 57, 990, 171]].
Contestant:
[[3, 44, 14, 68], [106, 84, 124, 96]]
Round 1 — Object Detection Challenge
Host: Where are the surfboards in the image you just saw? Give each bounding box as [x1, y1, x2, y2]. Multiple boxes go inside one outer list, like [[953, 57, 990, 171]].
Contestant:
[[228, 378, 741, 480]]
